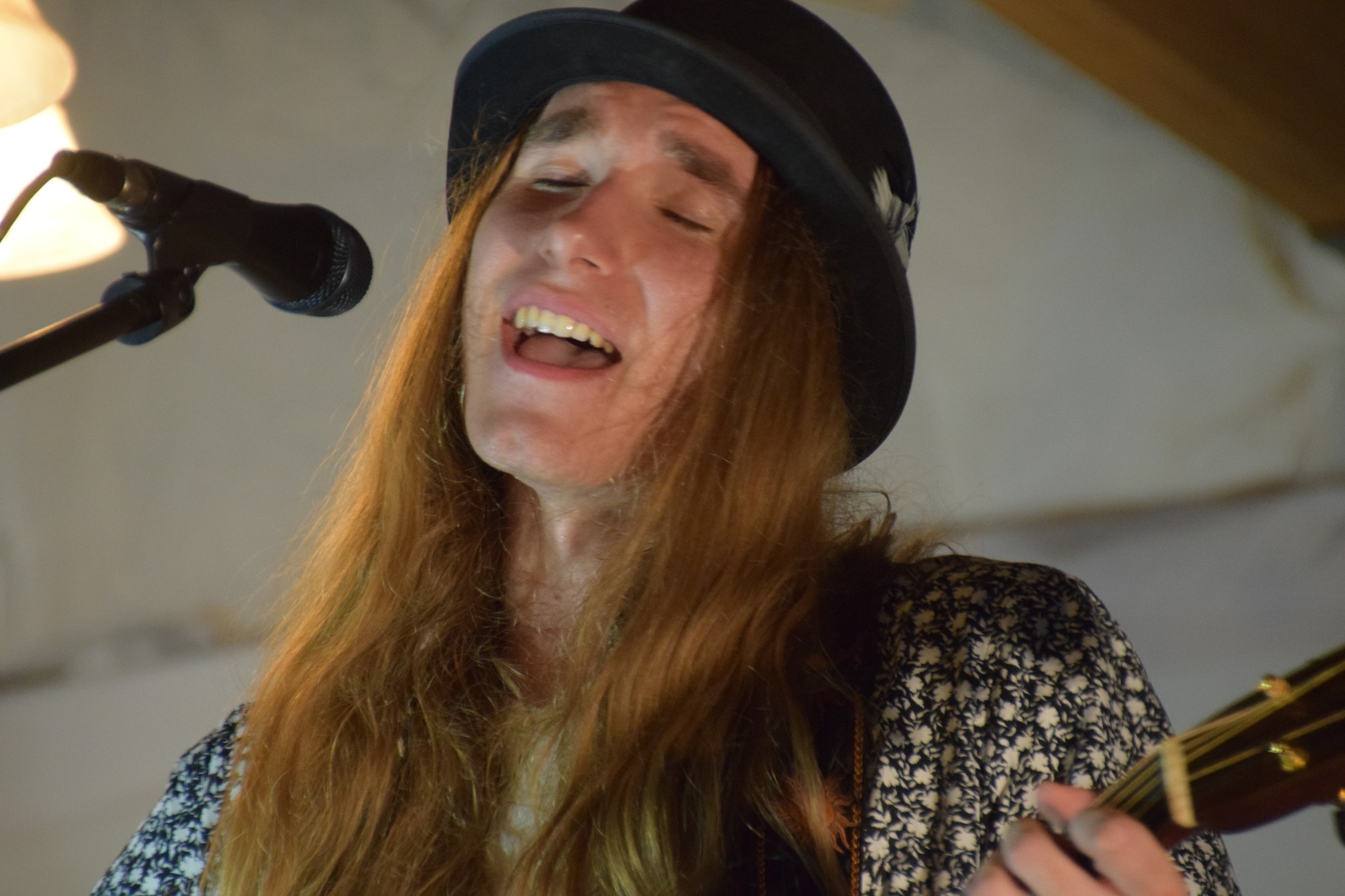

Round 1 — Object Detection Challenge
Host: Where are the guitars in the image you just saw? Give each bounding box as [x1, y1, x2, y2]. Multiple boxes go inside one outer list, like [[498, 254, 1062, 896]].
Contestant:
[[1099, 640, 1345, 896]]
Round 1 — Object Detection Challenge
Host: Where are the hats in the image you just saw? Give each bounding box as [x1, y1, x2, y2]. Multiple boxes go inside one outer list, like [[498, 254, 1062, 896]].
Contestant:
[[445, 0, 920, 475]]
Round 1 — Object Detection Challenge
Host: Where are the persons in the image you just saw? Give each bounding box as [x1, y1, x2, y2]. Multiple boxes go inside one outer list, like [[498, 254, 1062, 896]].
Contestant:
[[90, 0, 1241, 896]]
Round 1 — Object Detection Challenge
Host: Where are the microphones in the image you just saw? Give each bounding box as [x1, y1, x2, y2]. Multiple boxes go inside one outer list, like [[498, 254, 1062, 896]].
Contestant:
[[53, 149, 374, 317]]
